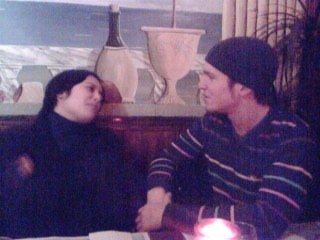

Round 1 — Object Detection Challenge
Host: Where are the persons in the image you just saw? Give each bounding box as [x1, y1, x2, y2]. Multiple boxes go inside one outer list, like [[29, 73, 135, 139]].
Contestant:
[[0, 69, 148, 239], [135, 37, 318, 240]]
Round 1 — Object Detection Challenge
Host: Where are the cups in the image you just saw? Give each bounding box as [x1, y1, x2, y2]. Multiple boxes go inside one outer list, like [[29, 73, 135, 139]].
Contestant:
[[232, 222, 258, 240]]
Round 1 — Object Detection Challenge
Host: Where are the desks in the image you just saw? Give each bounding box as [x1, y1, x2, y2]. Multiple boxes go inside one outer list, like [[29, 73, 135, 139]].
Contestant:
[[0, 222, 320, 240]]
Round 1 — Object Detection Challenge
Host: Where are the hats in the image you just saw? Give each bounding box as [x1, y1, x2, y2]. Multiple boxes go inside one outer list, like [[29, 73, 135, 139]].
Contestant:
[[205, 36, 279, 92]]
